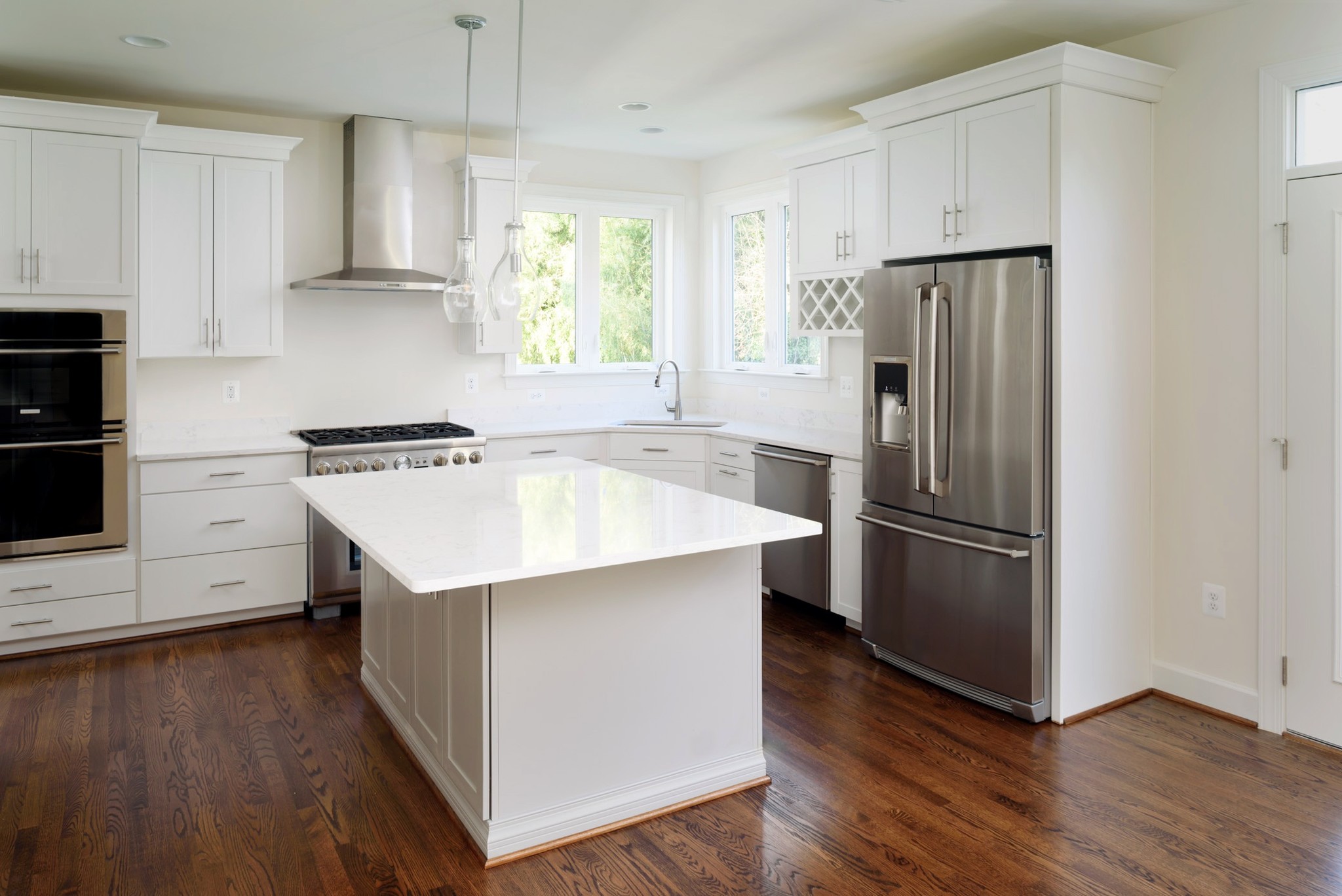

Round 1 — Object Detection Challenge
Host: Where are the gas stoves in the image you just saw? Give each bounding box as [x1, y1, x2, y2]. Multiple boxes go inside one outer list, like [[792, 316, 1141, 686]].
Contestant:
[[298, 419, 488, 475]]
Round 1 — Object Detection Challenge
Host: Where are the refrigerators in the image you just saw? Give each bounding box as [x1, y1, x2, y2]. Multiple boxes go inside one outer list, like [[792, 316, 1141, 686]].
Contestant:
[[856, 244, 1063, 726]]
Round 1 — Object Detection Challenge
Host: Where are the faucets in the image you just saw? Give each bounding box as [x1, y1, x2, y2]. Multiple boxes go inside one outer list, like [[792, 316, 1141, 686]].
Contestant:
[[653, 358, 683, 421]]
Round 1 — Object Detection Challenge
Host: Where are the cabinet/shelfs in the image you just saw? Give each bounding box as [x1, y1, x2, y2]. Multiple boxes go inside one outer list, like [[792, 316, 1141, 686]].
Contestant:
[[0, 96, 302, 358], [0, 446, 310, 655], [477, 424, 876, 631]]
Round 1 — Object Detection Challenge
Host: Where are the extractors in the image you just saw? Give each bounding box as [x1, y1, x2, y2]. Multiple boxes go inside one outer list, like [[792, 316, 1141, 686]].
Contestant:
[[292, 114, 467, 298]]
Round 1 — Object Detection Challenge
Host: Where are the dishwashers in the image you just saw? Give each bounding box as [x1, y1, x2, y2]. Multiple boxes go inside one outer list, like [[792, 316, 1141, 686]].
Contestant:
[[752, 438, 835, 613]]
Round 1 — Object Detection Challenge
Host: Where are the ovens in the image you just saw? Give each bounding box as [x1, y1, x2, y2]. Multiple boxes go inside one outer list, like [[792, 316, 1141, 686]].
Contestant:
[[0, 302, 136, 563], [305, 498, 364, 609]]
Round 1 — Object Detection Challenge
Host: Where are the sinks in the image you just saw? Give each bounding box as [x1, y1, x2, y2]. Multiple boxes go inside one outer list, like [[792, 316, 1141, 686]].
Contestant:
[[613, 419, 729, 431]]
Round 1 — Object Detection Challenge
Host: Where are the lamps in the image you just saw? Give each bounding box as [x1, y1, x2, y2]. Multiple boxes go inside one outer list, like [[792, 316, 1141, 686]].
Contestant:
[[446, 13, 492, 325], [483, 1, 546, 323]]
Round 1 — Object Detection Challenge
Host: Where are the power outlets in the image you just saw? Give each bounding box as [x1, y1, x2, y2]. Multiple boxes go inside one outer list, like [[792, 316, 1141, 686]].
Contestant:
[[1200, 582, 1227, 620], [221, 379, 238, 406], [463, 372, 479, 398]]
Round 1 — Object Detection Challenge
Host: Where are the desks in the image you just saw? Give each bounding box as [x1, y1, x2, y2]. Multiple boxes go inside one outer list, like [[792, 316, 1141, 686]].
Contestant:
[[289, 451, 823, 871]]
